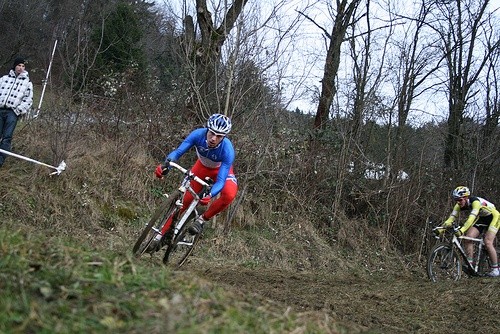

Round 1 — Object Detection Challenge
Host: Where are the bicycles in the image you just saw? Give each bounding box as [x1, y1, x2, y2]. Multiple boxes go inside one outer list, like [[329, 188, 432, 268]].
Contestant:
[[426, 222, 500, 284], [133, 158, 205, 272]]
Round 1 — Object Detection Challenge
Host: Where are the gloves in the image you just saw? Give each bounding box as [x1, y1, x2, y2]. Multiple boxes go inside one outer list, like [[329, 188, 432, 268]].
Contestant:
[[455, 230, 461, 237], [199, 195, 211, 206], [155, 164, 164, 179]]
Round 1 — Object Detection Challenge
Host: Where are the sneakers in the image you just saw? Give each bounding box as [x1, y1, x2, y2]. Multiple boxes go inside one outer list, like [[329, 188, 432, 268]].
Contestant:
[[144, 240, 158, 250], [188, 221, 203, 235]]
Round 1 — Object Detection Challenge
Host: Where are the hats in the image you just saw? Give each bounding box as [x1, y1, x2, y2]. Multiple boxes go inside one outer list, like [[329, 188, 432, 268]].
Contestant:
[[13, 57, 25, 69]]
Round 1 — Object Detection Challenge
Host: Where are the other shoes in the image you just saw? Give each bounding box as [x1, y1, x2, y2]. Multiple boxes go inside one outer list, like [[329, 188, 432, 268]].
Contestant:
[[485, 269, 499, 277]]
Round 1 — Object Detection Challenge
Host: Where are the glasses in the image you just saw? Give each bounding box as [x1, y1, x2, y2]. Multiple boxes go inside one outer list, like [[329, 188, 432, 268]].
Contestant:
[[455, 198, 465, 202], [208, 129, 225, 139]]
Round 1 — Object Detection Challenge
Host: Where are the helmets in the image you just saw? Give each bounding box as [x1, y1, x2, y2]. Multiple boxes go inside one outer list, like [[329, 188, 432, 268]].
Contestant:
[[453, 186, 470, 198], [207, 114, 231, 136]]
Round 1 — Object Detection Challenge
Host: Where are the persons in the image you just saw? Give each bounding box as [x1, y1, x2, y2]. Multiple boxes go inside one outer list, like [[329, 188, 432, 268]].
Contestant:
[[0, 58, 33, 168], [431, 186, 500, 276], [145, 114, 238, 254]]
